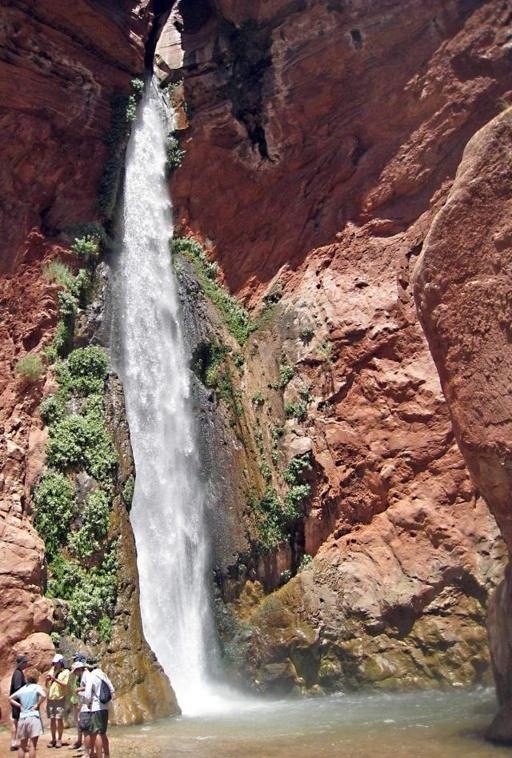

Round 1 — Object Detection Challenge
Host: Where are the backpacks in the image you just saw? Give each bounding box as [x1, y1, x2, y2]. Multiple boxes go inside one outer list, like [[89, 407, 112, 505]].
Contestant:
[[91, 673, 112, 705]]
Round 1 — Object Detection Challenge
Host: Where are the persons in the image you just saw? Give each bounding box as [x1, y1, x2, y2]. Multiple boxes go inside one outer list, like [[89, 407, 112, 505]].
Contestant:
[[8, 668, 47, 758], [45, 654, 70, 748], [78, 657, 116, 758], [69, 652, 87, 749], [9, 653, 31, 752], [69, 660, 96, 758]]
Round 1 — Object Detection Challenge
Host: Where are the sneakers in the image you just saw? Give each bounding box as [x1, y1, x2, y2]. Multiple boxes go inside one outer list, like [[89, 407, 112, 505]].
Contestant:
[[71, 741, 91, 758], [47, 739, 63, 748], [10, 742, 29, 752]]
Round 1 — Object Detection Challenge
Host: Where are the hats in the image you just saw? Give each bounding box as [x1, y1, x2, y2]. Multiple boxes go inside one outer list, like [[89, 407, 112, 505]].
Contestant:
[[51, 653, 65, 663], [15, 655, 28, 667], [85, 657, 99, 669], [71, 651, 89, 659], [68, 661, 85, 675]]
